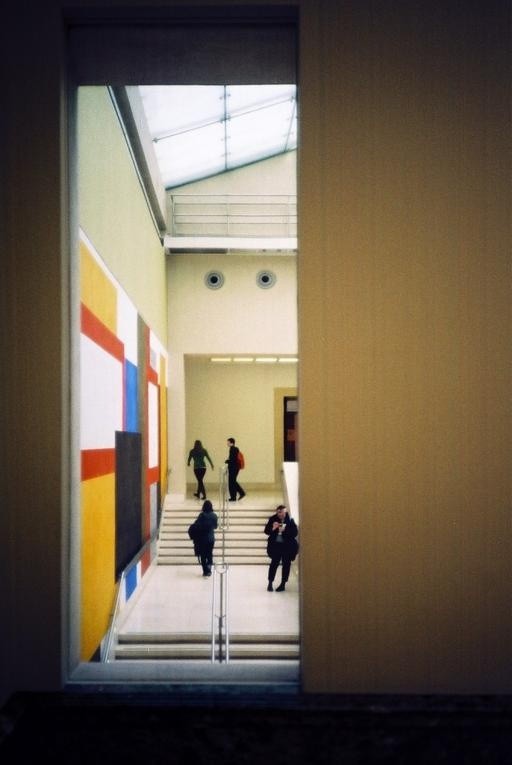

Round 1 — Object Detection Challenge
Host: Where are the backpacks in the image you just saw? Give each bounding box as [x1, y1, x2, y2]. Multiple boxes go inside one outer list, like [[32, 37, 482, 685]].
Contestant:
[[236, 452, 244, 469]]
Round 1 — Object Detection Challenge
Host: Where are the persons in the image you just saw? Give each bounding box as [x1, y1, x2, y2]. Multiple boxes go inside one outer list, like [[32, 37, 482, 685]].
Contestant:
[[264, 505, 299, 593], [225, 437, 245, 502], [188, 499, 218, 576], [186, 439, 214, 500]]
